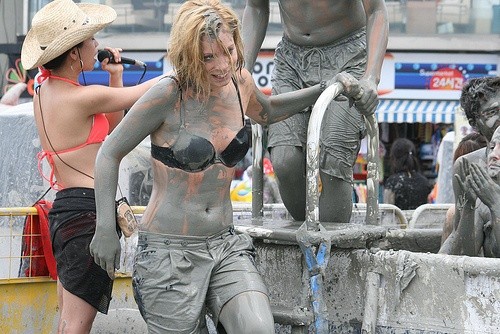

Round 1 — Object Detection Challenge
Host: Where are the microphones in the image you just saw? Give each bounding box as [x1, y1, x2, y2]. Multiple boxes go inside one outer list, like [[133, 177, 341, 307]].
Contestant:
[[97, 50, 147, 67]]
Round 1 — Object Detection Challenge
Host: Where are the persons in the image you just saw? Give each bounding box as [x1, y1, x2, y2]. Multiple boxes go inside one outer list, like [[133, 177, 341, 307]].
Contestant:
[[238, 0, 389, 223], [227, 74, 500, 261], [19, 1, 179, 334], [88, 0, 362, 334]]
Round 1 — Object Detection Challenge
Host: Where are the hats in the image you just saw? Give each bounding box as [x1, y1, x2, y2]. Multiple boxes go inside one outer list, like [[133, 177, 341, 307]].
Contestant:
[[20, 0, 118, 71]]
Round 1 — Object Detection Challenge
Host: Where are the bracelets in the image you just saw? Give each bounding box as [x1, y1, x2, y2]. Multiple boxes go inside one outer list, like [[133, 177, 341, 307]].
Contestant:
[[320, 80, 326, 93]]
